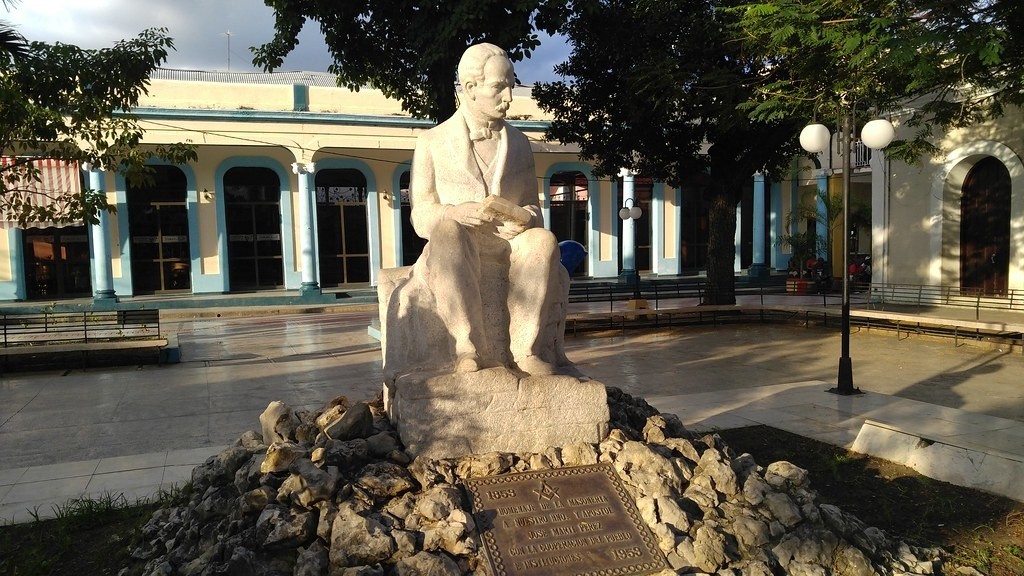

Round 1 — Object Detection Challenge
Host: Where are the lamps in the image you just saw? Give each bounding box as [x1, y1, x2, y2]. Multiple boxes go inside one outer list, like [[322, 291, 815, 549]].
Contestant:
[[202, 189, 214, 201], [382, 189, 391, 200]]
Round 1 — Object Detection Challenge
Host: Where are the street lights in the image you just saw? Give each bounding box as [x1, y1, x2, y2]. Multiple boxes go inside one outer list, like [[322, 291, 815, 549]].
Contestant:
[[799, 88, 894, 396], [618, 197, 653, 321]]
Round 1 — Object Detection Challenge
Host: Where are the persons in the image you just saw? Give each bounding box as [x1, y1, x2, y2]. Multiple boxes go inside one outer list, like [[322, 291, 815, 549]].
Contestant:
[[787, 251, 872, 296], [409, 41, 562, 378]]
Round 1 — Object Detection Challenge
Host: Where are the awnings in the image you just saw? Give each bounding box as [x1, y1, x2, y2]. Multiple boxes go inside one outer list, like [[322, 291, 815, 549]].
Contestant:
[[0, 152, 85, 230]]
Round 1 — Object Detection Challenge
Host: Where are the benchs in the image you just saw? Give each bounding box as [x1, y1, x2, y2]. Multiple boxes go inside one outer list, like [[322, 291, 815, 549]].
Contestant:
[[564, 276, 1024, 350], [1, 308, 173, 374]]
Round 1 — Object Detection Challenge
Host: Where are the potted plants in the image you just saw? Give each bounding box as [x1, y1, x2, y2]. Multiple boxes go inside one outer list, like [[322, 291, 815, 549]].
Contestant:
[[773, 232, 834, 295]]
